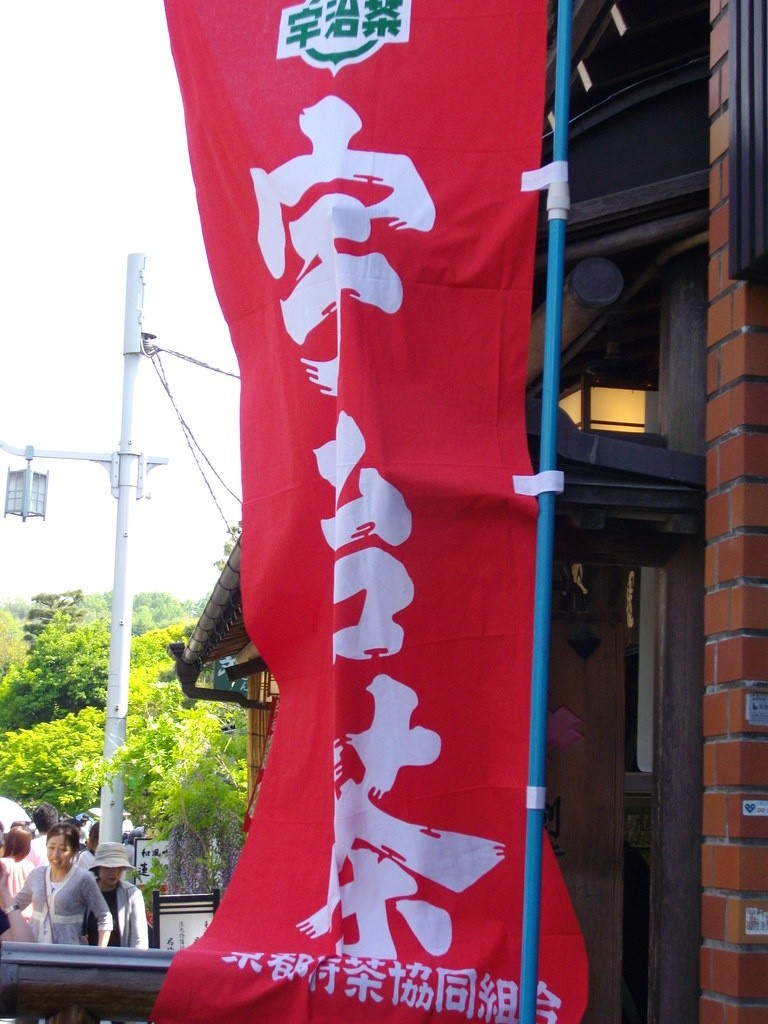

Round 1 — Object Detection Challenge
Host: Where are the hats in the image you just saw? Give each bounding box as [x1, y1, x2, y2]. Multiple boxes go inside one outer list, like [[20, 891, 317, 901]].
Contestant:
[[88, 841, 136, 872]]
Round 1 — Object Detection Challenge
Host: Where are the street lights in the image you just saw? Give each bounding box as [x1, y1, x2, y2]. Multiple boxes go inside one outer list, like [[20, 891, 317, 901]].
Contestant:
[[0, 433, 171, 845]]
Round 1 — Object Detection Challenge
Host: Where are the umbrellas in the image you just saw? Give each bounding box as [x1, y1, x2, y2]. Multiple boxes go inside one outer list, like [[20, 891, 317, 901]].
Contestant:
[[0, 797, 31, 833], [89, 808, 101, 817]]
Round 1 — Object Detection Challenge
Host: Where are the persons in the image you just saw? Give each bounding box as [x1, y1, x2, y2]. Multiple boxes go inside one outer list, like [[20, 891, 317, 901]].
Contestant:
[[0, 804, 149, 1024]]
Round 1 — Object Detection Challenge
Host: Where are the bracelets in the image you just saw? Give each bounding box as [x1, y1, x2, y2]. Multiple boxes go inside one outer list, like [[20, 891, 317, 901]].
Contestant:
[[5, 905, 20, 915]]
